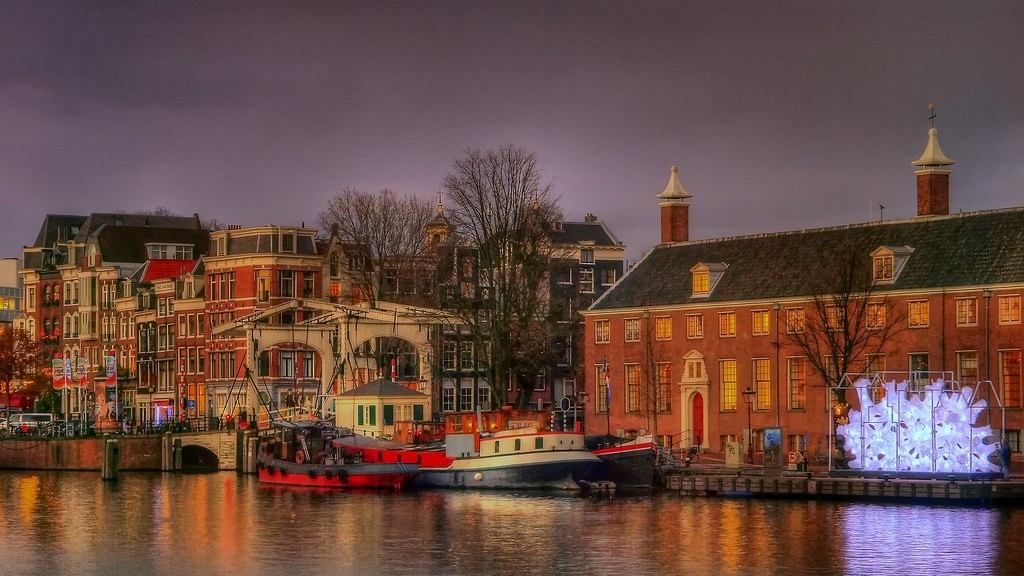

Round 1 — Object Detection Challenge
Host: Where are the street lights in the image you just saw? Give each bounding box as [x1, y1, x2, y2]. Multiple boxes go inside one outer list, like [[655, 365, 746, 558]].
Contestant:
[[742, 386, 758, 464]]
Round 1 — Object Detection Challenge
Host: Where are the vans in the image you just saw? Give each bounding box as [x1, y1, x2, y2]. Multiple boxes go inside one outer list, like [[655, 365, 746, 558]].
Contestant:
[[0, 412, 62, 436]]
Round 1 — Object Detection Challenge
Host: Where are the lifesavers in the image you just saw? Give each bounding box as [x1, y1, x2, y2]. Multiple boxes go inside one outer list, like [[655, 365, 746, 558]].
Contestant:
[[295, 451, 304, 464], [239, 422, 248, 430], [310, 414, 315, 420], [224, 412, 232, 422]]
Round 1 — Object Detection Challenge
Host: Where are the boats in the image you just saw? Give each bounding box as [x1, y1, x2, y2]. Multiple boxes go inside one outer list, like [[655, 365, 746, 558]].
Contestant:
[[590, 432, 658, 498], [332, 406, 604, 491], [256, 417, 419, 490]]
